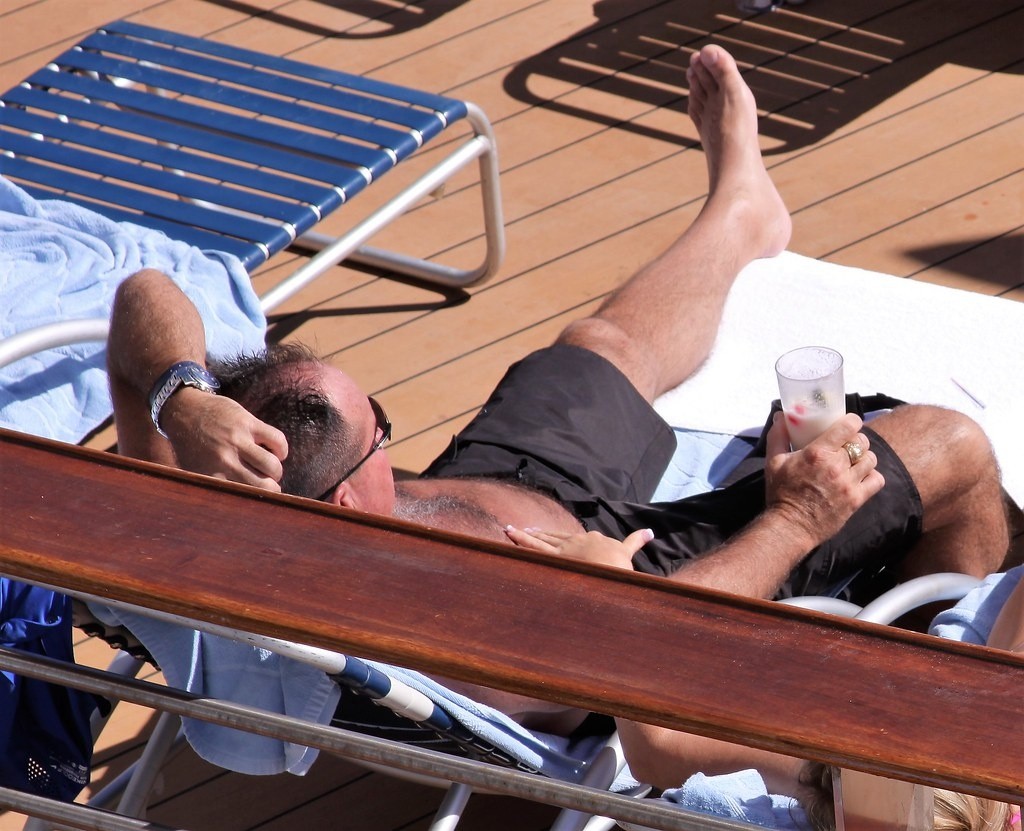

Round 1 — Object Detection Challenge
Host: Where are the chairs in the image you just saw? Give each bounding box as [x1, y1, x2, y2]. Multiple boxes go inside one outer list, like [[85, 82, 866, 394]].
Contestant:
[[0, 252, 1024, 831], [0, 18, 505, 451]]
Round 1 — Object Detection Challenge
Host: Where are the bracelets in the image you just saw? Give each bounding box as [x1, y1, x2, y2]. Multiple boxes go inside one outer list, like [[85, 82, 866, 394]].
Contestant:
[[149, 360, 200, 406]]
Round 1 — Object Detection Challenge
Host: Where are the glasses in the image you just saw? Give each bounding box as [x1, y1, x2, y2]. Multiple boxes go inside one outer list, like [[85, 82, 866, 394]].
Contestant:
[[318, 395, 394, 501]]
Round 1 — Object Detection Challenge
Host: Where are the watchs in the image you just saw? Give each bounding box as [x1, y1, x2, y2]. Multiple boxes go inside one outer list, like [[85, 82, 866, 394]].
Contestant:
[[151, 366, 222, 440]]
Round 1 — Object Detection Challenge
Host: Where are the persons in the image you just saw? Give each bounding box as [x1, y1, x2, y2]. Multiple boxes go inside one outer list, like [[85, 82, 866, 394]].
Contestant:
[[106, 43, 1010, 736], [505, 524, 1024, 831]]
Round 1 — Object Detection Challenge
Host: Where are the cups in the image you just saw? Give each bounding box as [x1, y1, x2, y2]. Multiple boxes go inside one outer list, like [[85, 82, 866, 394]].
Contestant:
[[774, 346, 846, 452]]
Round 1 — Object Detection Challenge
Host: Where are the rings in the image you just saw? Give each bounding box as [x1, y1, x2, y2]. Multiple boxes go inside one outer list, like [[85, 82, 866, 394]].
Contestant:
[[842, 442, 866, 465]]
